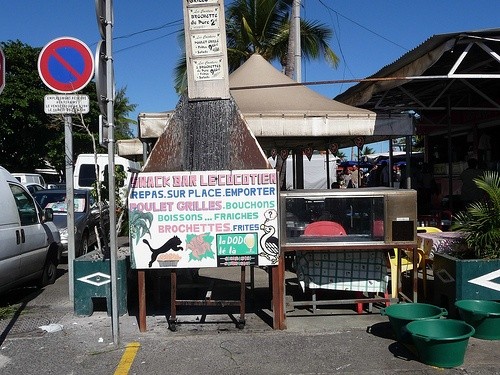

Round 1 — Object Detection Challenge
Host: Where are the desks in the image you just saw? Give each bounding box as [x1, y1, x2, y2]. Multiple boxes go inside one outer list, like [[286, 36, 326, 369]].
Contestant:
[[417, 232, 469, 260]]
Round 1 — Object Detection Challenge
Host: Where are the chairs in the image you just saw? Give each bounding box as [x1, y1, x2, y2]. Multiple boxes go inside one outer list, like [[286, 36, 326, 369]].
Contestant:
[[406, 227, 443, 298]]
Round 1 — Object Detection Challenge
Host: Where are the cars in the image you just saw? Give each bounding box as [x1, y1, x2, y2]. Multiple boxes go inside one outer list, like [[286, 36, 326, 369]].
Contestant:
[[25, 181, 57, 196], [31, 189, 110, 257]]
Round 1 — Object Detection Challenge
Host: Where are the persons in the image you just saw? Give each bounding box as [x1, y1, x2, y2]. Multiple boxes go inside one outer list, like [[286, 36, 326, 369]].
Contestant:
[[460, 158, 478, 206], [331, 161, 406, 189]]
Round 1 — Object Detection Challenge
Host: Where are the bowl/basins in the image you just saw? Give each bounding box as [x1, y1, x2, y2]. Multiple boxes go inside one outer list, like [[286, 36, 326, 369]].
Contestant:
[[455, 299, 500, 340], [382, 303, 448, 337], [406, 319, 475, 367]]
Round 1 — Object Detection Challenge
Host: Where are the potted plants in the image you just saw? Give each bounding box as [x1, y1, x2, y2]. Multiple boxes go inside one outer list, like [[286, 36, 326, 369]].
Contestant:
[[434, 170, 500, 319]]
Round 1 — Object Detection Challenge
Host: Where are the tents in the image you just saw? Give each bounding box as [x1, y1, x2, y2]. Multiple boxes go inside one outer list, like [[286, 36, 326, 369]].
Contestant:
[[115, 52, 414, 189]]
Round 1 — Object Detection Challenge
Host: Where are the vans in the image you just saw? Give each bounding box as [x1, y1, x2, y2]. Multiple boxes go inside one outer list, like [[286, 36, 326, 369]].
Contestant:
[[0, 166, 61, 293], [73, 153, 142, 208], [8, 172, 46, 189]]
